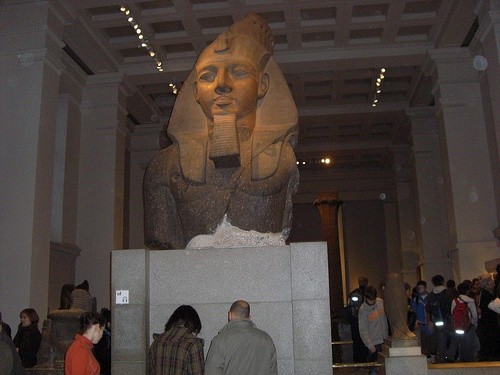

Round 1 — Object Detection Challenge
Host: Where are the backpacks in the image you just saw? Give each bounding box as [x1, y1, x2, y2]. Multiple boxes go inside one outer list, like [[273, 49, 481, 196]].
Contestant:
[[451, 298, 470, 330], [350, 289, 364, 316]]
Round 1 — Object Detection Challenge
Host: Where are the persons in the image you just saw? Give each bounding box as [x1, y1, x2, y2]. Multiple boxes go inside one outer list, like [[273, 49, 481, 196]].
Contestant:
[[147, 305, 204, 375], [0, 307, 111, 375], [140, 14, 299, 248], [348, 274, 500, 375], [204, 300, 278, 375]]
